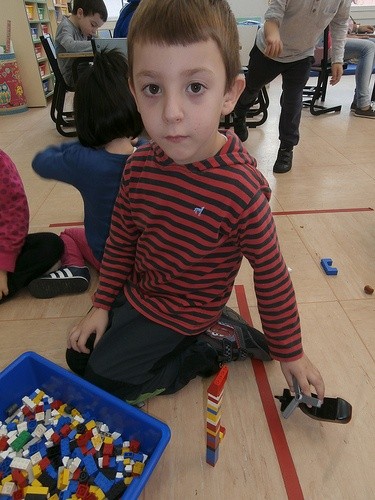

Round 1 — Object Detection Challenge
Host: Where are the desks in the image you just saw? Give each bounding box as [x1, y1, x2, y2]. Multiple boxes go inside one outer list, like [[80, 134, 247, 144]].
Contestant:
[[58, 52, 94, 59]]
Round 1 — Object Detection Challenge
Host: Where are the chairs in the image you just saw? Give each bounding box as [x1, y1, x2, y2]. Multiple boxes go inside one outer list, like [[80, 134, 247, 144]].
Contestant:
[[91, 37, 128, 61], [39, 33, 78, 137], [216, 20, 348, 129]]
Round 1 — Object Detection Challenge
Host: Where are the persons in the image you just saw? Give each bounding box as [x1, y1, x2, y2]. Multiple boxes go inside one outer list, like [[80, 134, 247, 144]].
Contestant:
[[233, 1, 352, 174], [29, 51, 148, 299], [65, 0, 325, 408], [0, 149, 65, 304], [54, 0, 108, 88], [312, 23, 375, 119]]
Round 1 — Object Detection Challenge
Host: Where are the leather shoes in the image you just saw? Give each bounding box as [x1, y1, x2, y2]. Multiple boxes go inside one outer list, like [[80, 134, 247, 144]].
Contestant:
[[233, 110, 248, 142], [273, 148, 293, 173]]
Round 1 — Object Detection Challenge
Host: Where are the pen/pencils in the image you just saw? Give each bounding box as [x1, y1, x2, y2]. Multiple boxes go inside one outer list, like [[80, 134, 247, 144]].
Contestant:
[[109, 30, 112, 38]]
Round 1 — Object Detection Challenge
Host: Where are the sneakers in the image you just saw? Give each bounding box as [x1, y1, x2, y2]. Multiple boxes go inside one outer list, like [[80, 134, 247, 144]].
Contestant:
[[350, 102, 356, 112], [29, 265, 90, 299], [354, 104, 375, 118], [194, 305, 272, 363]]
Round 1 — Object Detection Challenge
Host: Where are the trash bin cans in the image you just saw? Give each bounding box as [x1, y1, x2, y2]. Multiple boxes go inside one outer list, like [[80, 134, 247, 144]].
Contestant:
[[0, 53, 28, 116]]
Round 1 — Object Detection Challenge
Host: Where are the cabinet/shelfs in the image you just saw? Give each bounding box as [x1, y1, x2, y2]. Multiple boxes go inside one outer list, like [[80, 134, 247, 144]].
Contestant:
[[0, 0, 73, 108]]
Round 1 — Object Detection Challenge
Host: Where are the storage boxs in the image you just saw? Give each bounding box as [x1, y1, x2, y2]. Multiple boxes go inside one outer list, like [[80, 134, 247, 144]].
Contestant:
[[0, 351, 171, 500], [0, 52, 29, 115]]
